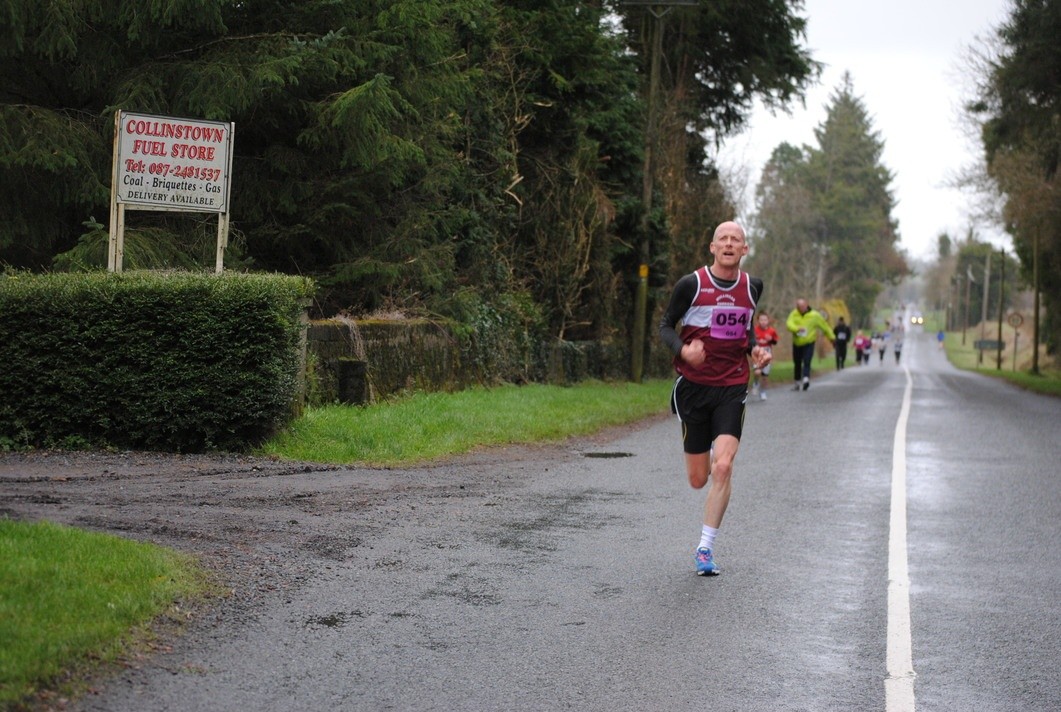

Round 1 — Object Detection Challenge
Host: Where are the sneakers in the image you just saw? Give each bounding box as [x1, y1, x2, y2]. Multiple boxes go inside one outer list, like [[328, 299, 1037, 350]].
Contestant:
[[760, 391, 767, 400], [695, 546, 720, 575], [795, 379, 800, 390], [753, 383, 760, 395], [803, 376, 809, 390]]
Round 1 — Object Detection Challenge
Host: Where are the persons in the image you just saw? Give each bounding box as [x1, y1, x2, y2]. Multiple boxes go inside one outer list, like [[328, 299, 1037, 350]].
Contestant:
[[785, 298, 837, 393], [863, 327, 904, 366], [937, 328, 945, 350], [851, 330, 867, 368], [657, 221, 772, 577], [750, 309, 780, 402], [830, 317, 853, 371]]
